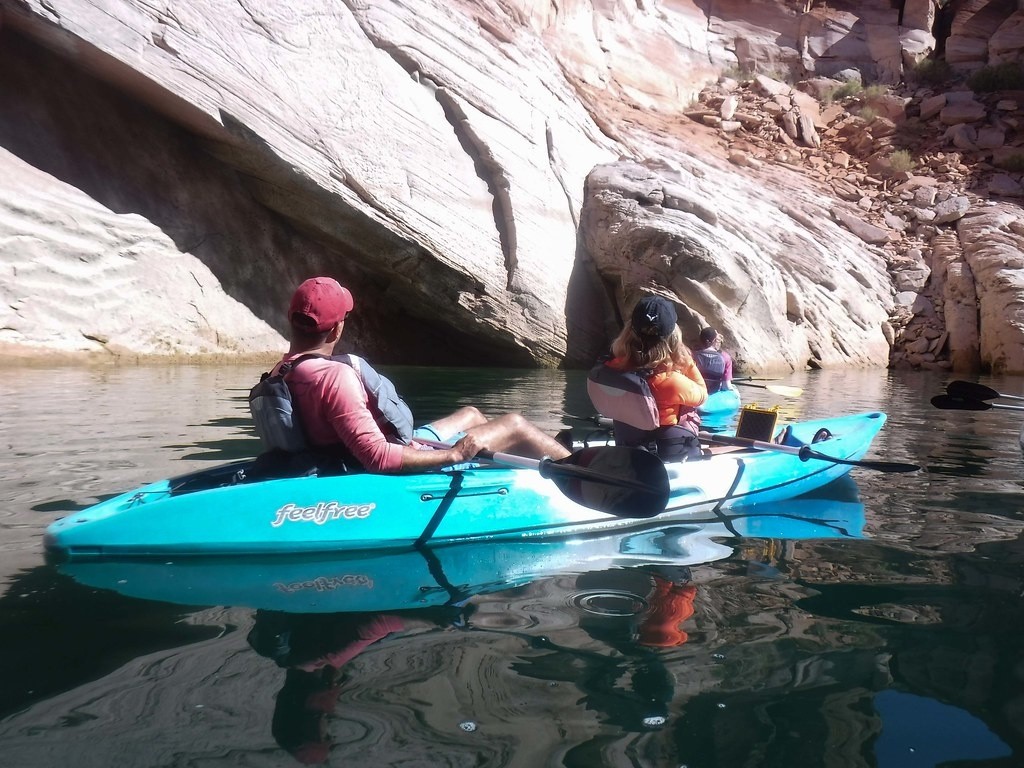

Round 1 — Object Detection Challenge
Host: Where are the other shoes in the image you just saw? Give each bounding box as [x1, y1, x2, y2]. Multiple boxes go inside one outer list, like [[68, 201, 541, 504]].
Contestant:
[[554, 430, 573, 453]]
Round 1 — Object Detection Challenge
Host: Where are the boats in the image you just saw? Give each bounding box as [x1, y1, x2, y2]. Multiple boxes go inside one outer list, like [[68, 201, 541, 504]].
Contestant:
[[43, 409, 888, 561], [47, 466, 866, 613]]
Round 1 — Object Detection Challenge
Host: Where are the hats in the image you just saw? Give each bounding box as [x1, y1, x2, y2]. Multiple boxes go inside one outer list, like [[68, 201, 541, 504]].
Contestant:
[[701, 327, 716, 343], [288, 277, 353, 332], [632, 296, 676, 341]]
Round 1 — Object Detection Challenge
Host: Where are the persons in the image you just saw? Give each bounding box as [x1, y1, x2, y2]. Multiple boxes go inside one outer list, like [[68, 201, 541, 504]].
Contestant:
[[690, 326, 735, 396], [574, 566, 696, 704], [245, 610, 454, 763], [249, 277, 571, 475], [604, 295, 832, 463]]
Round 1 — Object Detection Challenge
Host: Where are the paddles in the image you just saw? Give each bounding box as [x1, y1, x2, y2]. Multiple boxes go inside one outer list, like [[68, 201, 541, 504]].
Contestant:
[[413, 436, 669, 518], [548, 408, 920, 474], [931, 394, 1024, 411], [730, 381, 804, 398], [731, 375, 784, 381], [946, 381, 1024, 402]]
